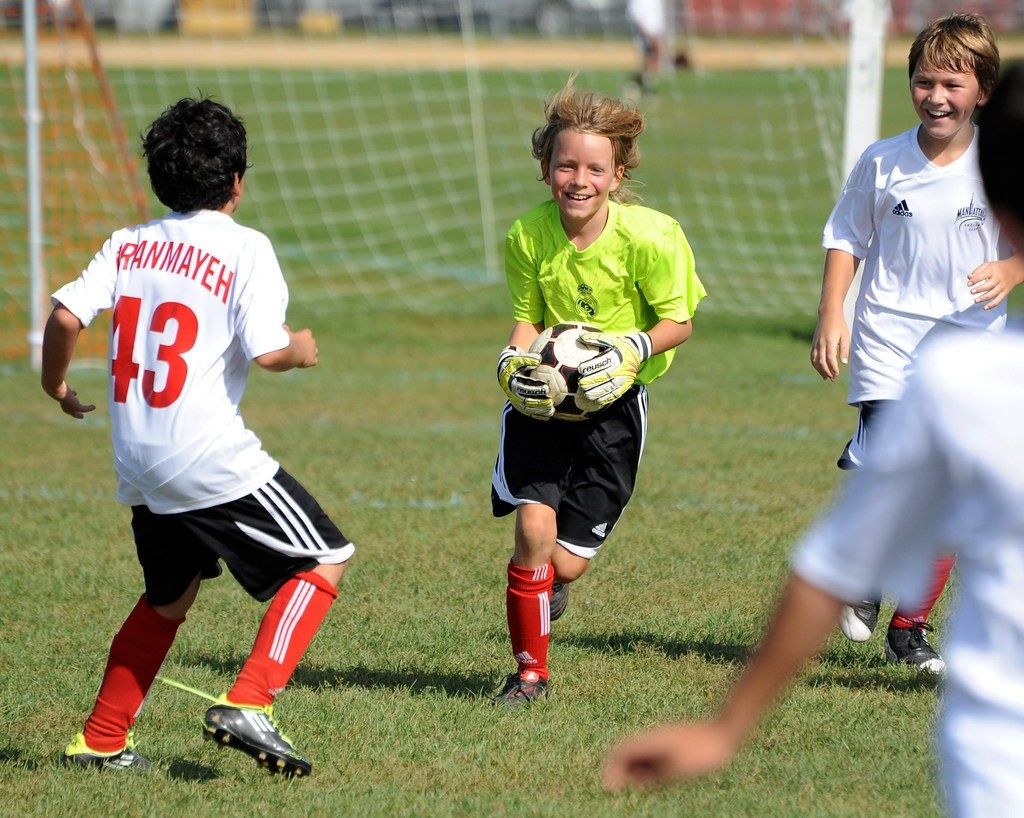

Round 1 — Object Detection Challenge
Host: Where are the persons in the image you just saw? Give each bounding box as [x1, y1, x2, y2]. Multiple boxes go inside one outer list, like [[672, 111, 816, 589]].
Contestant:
[[40, 100, 356, 778], [809, 13, 1024, 670], [602, 59, 1024, 818], [491, 90, 707, 697]]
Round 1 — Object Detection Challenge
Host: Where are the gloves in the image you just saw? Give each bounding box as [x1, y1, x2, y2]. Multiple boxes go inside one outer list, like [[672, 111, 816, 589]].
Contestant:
[[578, 328, 652, 405], [497, 345, 555, 420]]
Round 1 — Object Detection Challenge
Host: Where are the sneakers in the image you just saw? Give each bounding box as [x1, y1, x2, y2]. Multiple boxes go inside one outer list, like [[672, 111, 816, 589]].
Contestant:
[[837, 587, 882, 642], [549, 576, 568, 620], [62, 725, 156, 775], [884, 622, 946, 674], [202, 695, 312, 777], [492, 669, 548, 707]]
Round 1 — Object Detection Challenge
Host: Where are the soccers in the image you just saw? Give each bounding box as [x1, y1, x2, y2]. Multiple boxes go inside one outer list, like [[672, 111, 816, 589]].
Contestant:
[[526, 320, 614, 424]]
